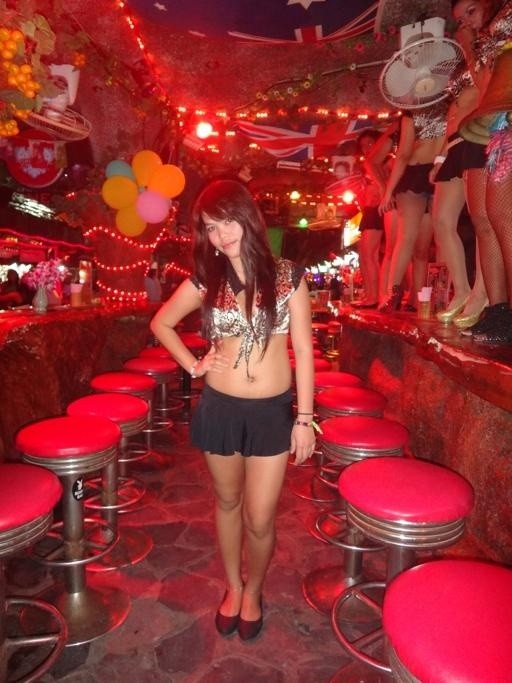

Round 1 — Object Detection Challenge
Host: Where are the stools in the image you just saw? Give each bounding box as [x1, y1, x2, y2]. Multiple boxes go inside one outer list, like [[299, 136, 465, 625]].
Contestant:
[[287, 332, 332, 468], [1, 458, 72, 682], [139, 317, 212, 427], [14, 412, 135, 650], [329, 456, 511, 683], [68, 391, 153, 573], [122, 358, 181, 472], [293, 371, 365, 504], [314, 386, 387, 548], [300, 413, 411, 620], [88, 371, 158, 512]]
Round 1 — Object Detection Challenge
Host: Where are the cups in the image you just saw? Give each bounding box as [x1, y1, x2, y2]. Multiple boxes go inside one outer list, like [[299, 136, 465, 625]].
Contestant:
[[417, 300, 430, 319], [71, 292, 82, 306]]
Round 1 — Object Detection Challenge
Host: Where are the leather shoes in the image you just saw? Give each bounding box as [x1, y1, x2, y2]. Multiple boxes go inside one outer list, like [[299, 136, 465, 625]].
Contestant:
[[351, 288, 420, 314], [216, 578, 245, 636], [239, 586, 262, 639], [436, 292, 512, 341]]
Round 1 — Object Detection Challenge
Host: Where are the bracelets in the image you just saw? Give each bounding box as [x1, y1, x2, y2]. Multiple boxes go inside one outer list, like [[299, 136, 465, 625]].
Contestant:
[[190, 358, 201, 379], [295, 411, 315, 416], [293, 420, 317, 428]]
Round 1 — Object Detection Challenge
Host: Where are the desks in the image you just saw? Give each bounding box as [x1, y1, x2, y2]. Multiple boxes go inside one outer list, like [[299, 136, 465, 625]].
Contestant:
[[1, 287, 173, 450], [328, 288, 509, 558]]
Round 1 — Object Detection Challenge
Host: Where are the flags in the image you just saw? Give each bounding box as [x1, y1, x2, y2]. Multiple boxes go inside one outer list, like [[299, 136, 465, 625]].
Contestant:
[[230, 113, 393, 164]]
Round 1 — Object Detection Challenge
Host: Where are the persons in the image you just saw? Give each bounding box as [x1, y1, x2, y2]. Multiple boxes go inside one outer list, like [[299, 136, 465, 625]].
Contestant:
[[238, 159, 254, 183], [335, 159, 353, 184], [0, 268, 32, 310], [151, 177, 316, 641], [145, 263, 168, 302], [349, 0, 511, 347]]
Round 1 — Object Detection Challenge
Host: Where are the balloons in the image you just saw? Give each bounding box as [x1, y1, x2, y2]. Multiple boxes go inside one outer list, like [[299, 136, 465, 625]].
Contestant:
[[100, 149, 187, 238]]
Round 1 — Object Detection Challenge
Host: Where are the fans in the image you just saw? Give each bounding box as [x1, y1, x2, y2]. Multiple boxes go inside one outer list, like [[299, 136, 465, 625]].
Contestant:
[[11, 64, 96, 142], [377, 17, 472, 108]]
